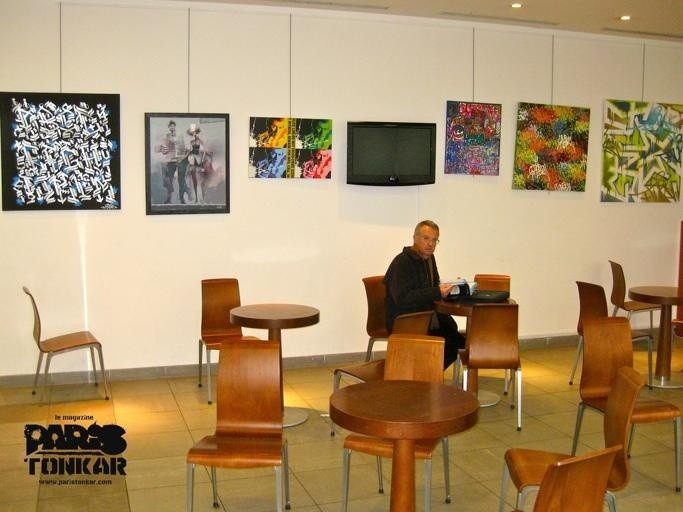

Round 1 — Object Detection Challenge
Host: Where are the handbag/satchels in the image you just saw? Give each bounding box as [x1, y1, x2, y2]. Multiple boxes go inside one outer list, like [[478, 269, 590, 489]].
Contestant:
[[441, 287, 512, 306]]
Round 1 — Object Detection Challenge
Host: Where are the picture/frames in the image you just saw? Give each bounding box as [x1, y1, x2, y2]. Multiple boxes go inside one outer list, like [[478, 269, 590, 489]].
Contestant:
[[144, 112, 231, 215]]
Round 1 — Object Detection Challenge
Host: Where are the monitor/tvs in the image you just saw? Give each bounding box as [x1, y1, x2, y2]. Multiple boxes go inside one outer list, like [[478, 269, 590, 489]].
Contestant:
[[346, 121, 436, 186]]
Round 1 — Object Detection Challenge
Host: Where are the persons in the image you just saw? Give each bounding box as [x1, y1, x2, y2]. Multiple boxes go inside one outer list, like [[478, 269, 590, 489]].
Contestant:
[[154, 121, 186, 203], [383, 220, 465, 369], [255, 118, 331, 179], [177, 123, 215, 203]]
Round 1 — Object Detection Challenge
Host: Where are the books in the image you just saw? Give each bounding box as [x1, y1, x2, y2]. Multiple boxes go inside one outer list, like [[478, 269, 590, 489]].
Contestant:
[[440, 280, 478, 301]]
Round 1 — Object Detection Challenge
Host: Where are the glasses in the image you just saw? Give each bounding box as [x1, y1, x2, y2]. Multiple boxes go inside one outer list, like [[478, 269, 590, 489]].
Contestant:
[[417, 235, 441, 245]]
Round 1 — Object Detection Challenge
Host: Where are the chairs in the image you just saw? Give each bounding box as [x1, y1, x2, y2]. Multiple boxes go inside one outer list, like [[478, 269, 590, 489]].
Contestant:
[[185, 341, 292, 512], [328, 311, 433, 439], [197, 278, 259, 404], [458, 305, 524, 431], [453, 274, 512, 395], [341, 332, 451, 511], [359, 275, 395, 362], [23, 287, 110, 406]]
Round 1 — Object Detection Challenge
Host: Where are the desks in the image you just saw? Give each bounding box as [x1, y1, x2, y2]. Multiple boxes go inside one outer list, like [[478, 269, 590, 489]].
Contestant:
[[223, 303, 319, 430], [629, 286, 683, 390], [435, 295, 516, 409], [327, 379, 479, 512]]
